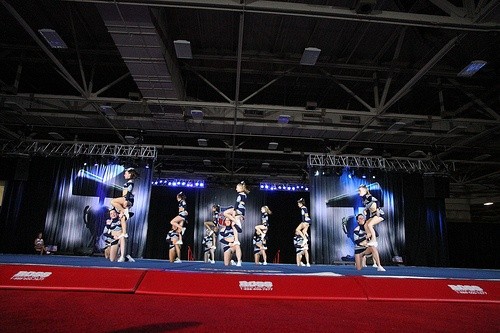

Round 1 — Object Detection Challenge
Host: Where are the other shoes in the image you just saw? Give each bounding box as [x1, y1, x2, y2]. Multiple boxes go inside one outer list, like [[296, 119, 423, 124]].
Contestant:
[[236, 219, 241, 228], [176, 241, 183, 244], [233, 241, 240, 244], [209, 231, 214, 236], [182, 227, 186, 235], [301, 262, 304, 266], [174, 259, 182, 263], [46, 251, 50, 254], [307, 263, 311, 267], [118, 257, 124, 262], [126, 255, 135, 262], [373, 264, 377, 267], [236, 262, 241, 266], [211, 261, 215, 263], [302, 239, 308, 244], [230, 260, 235, 265], [378, 266, 386, 272], [262, 234, 266, 238], [211, 246, 216, 249], [124, 208, 129, 219], [263, 262, 267, 265], [121, 233, 128, 237], [368, 241, 377, 246]]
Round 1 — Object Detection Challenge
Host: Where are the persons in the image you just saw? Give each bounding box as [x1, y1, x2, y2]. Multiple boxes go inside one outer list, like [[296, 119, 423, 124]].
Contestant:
[[34, 232, 50, 255], [219, 216, 242, 266], [294, 231, 310, 267], [170, 192, 188, 244], [357, 184, 385, 247], [223, 181, 250, 247], [110, 168, 139, 239], [202, 229, 215, 264], [204, 204, 220, 249], [296, 198, 311, 247], [254, 206, 272, 240], [353, 213, 385, 271], [252, 229, 267, 265], [166, 225, 181, 262], [103, 209, 135, 263]]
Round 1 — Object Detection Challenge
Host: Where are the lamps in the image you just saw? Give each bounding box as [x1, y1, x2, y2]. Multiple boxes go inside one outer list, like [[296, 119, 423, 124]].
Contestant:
[[473, 153, 491, 161], [203, 160, 211, 166], [299, 47, 321, 66], [4, 102, 26, 112], [99, 106, 117, 116], [278, 115, 291, 124], [48, 133, 64, 140], [359, 148, 372, 154], [125, 136, 135, 145], [261, 163, 270, 169], [174, 40, 192, 59], [38, 29, 68, 49], [457, 61, 487, 78], [191, 110, 203, 120], [268, 142, 278, 149], [198, 139, 207, 146]]
